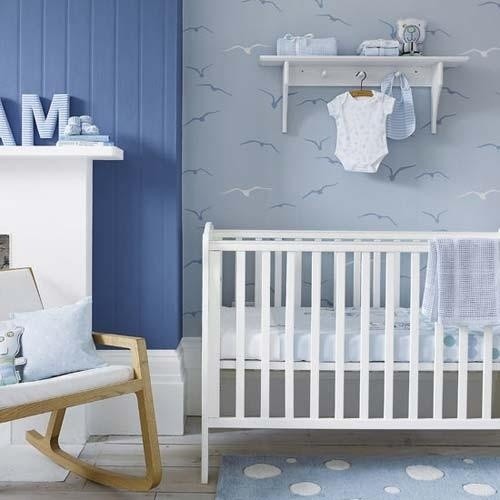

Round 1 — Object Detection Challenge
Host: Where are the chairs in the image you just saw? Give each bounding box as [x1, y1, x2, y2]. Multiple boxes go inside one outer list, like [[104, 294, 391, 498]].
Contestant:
[[0, 267, 163, 492]]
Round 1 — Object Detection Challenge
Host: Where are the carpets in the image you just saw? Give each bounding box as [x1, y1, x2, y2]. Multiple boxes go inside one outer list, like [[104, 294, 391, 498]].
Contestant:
[[214, 452, 500, 500]]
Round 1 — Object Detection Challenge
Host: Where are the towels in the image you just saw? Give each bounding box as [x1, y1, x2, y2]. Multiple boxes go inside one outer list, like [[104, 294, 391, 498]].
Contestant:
[[422, 237, 499, 326]]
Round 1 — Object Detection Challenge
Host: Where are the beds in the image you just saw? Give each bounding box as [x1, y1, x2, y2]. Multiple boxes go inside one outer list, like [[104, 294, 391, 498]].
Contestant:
[[201, 221, 500, 485]]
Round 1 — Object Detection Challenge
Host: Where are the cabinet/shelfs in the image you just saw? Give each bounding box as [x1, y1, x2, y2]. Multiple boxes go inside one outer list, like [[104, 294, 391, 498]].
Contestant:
[[351, 69, 375, 98]]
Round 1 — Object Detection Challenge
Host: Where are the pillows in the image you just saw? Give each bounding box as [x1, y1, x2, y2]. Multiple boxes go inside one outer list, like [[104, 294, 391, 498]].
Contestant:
[[11, 294, 110, 384]]
